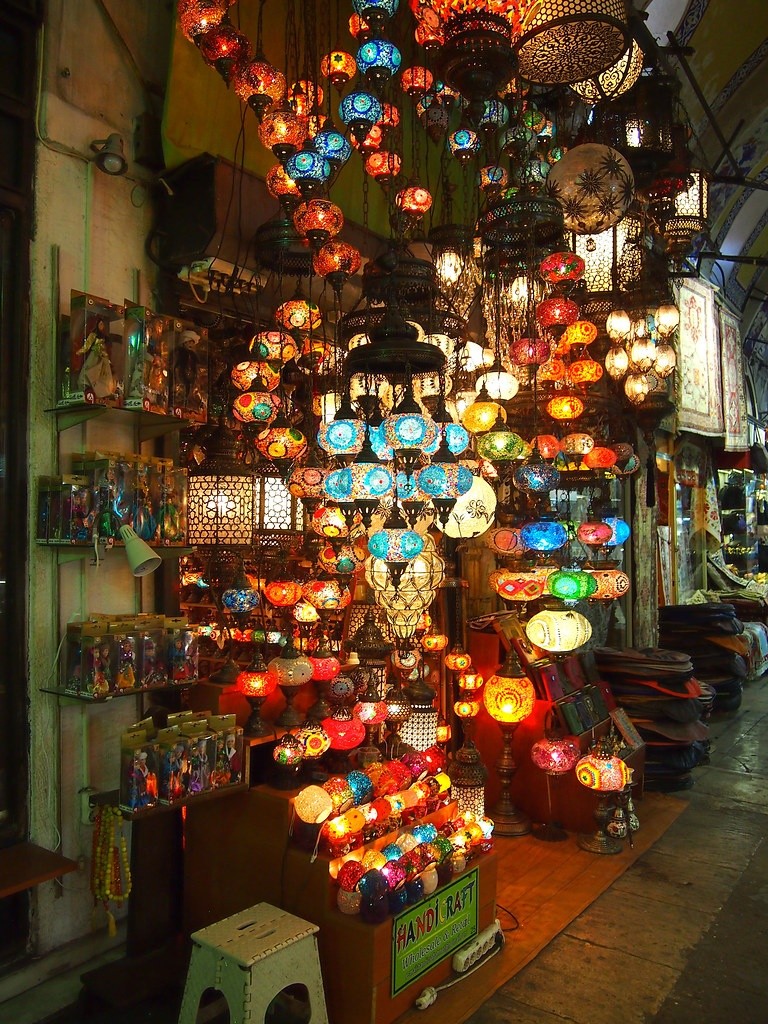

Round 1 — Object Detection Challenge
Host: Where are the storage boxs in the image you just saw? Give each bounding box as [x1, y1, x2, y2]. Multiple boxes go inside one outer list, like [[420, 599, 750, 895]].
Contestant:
[[38, 288, 244, 814]]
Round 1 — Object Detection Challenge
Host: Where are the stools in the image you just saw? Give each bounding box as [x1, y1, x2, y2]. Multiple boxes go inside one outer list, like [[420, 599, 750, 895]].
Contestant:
[[179, 902, 328, 1024]]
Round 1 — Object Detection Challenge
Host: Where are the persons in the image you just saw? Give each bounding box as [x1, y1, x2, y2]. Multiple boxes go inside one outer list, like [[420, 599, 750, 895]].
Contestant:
[[38, 288, 246, 806]]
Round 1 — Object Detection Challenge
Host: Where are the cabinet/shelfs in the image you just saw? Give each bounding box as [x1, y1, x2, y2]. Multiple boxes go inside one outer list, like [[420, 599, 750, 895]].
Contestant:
[[39, 246, 251, 823]]
